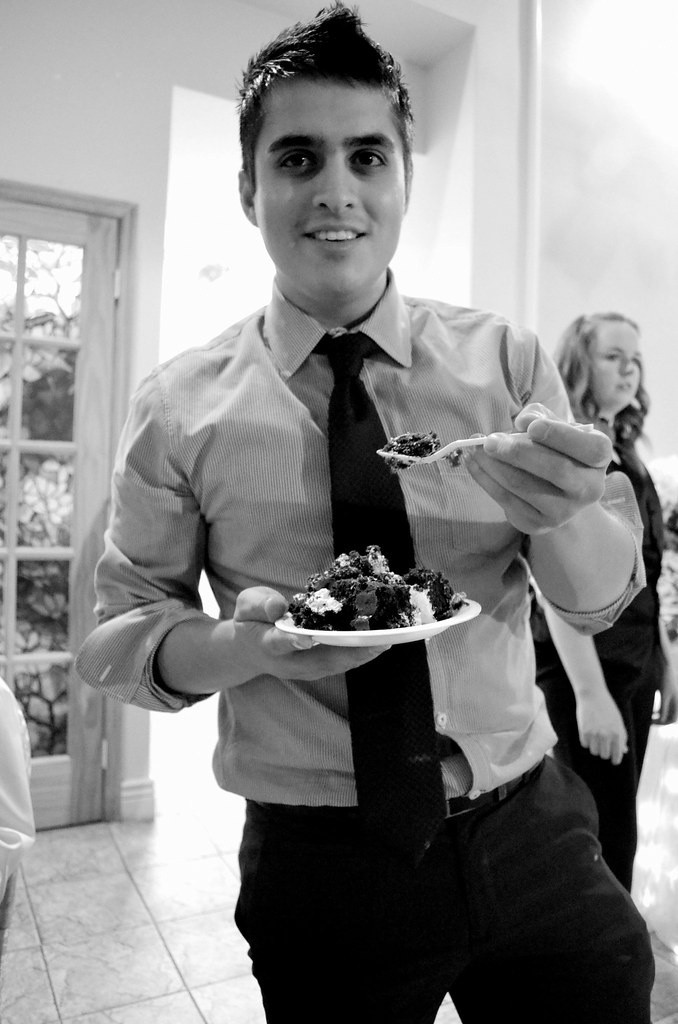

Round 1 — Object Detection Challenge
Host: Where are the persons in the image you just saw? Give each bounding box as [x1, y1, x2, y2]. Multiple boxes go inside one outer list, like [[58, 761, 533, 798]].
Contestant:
[[1, 679, 35, 897], [528, 313, 678, 898], [75, 0, 654, 1024]]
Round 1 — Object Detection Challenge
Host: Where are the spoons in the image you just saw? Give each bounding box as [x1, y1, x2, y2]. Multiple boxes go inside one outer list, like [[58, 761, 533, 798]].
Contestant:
[[375, 423, 594, 465]]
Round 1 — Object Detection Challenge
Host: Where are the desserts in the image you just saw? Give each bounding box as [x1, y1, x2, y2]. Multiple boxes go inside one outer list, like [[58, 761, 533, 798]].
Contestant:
[[287, 545, 466, 631], [381, 428, 440, 471]]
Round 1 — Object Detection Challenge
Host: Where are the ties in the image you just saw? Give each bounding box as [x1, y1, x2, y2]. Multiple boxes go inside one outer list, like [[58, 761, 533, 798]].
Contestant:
[[309, 330, 448, 881]]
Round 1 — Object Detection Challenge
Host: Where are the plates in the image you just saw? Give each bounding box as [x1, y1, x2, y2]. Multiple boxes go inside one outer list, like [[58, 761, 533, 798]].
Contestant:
[[273, 599, 482, 648]]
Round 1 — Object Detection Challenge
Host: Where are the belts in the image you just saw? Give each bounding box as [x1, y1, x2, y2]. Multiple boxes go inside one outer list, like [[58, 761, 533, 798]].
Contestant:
[[255, 758, 541, 825]]
